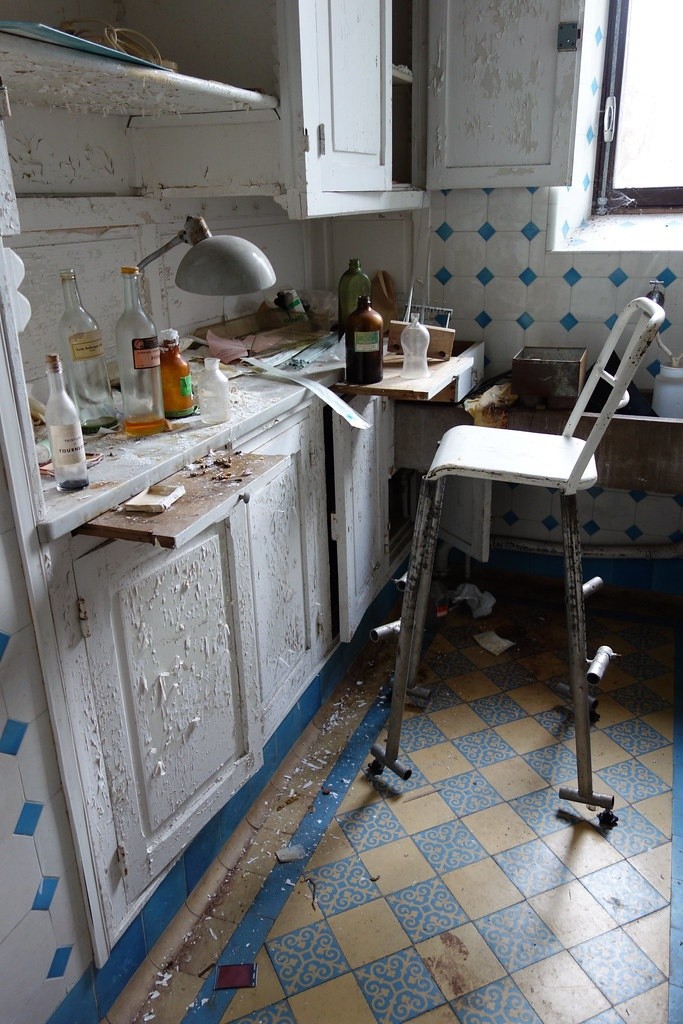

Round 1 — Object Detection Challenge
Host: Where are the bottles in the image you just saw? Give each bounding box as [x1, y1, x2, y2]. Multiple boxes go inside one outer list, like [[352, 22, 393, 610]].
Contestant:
[[339, 295, 385, 381], [336, 257, 371, 337], [199, 359, 230, 424], [42, 354, 91, 493], [151, 319, 193, 417], [115, 266, 165, 437], [53, 268, 114, 435], [401, 313, 429, 381]]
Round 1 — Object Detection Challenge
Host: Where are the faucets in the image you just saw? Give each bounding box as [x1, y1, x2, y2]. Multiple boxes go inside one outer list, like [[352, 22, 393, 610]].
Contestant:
[[647, 280, 664, 311]]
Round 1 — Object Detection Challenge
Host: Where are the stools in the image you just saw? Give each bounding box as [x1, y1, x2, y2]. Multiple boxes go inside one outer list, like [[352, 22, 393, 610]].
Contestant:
[[369, 296, 668, 828]]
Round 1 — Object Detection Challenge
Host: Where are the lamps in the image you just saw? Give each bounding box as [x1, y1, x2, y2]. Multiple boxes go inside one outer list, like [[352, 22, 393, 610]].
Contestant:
[[137, 216, 277, 297]]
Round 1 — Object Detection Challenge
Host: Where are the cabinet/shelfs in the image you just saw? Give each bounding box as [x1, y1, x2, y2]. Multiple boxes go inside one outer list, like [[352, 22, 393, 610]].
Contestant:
[[322, 334, 496, 671], [118, 0, 586, 219], [16, 357, 326, 953]]
